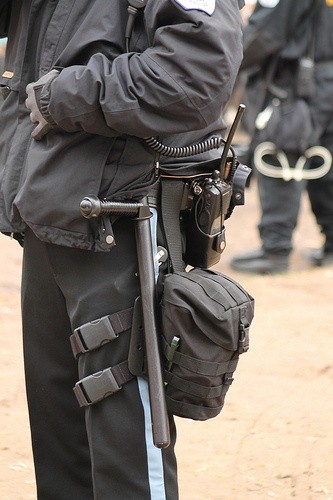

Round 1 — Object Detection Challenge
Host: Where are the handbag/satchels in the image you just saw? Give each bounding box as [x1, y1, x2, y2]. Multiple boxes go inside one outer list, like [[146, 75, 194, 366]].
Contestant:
[[150, 177, 255, 422]]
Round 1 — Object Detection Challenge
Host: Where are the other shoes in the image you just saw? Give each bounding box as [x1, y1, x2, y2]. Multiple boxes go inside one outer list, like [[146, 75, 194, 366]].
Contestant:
[[307, 249, 333, 267], [230, 251, 291, 273]]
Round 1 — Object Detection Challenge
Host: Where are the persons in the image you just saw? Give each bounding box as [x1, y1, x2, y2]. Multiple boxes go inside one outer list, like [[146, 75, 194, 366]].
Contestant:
[[242, 1, 333, 273], [1, 1, 242, 500]]
